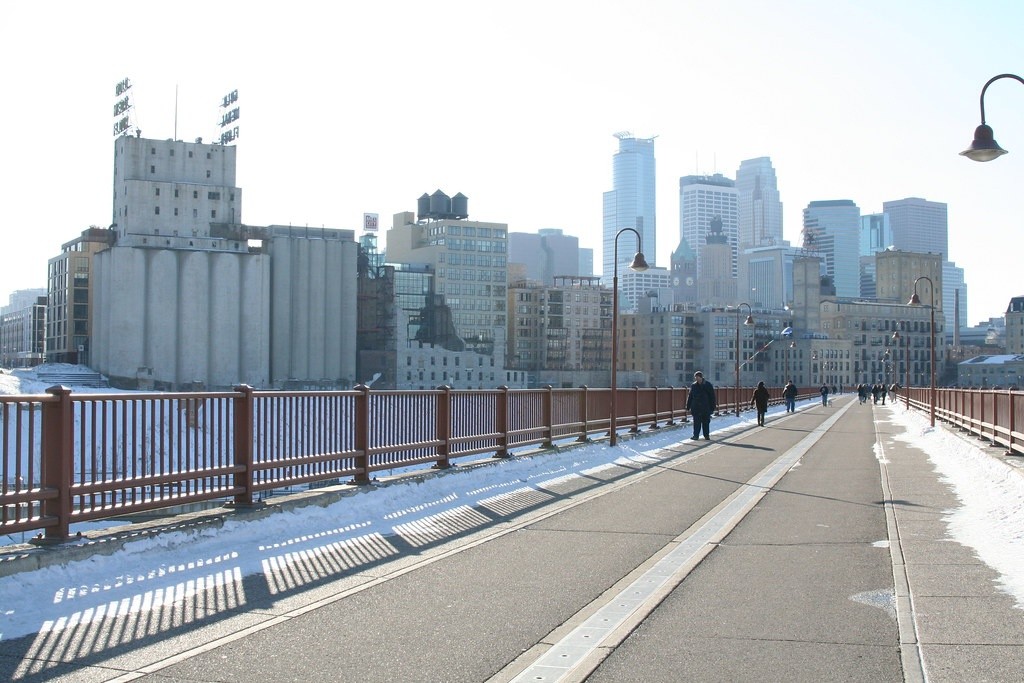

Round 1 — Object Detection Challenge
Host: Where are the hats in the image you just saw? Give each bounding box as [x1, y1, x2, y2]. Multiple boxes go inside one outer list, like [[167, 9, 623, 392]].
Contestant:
[[758, 381, 764, 385], [822, 383, 826, 385]]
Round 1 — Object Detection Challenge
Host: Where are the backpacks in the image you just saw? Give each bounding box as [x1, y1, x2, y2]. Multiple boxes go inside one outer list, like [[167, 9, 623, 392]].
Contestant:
[[821, 388, 825, 394]]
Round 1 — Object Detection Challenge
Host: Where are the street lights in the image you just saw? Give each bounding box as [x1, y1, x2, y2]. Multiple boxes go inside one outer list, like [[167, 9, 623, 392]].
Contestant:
[[784, 333, 797, 407], [906, 275, 937, 427], [880, 343, 897, 402], [736, 303, 756, 418], [891, 320, 910, 410], [607, 228, 650, 448], [809, 346, 817, 401]]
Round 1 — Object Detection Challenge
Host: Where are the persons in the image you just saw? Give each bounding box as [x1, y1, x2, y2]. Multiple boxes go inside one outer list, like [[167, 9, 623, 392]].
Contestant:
[[831, 382, 904, 405], [685, 371, 716, 440], [782, 380, 797, 412], [751, 381, 770, 427], [819, 383, 829, 407]]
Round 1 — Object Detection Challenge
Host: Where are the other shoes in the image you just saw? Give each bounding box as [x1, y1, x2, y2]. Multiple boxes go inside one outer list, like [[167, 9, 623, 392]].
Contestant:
[[690, 435, 699, 440], [705, 436, 710, 440]]
